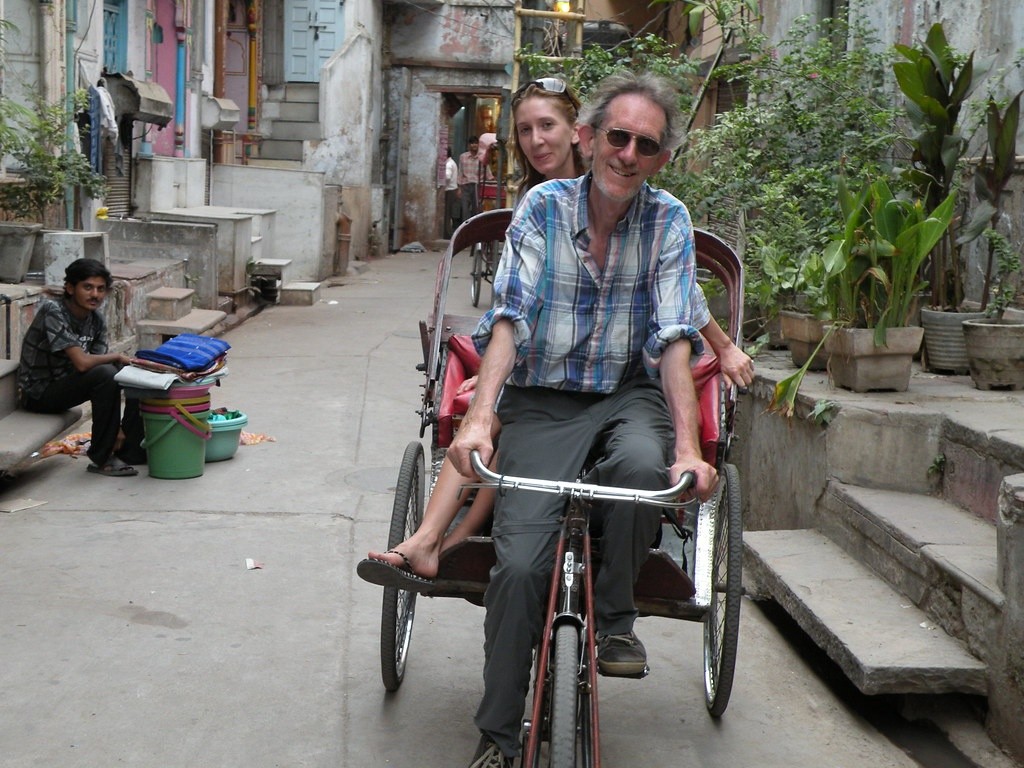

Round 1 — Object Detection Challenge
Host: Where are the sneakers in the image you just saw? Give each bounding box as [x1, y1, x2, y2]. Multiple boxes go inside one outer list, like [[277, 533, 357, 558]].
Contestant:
[[470, 734, 514, 768], [597, 630, 647, 675]]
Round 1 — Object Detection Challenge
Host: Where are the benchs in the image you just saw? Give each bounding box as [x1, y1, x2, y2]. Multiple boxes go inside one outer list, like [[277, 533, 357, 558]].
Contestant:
[[435, 333, 723, 467]]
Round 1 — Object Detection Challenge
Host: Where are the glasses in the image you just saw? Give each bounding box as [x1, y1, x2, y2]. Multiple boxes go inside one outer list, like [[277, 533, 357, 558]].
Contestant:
[[595, 127, 665, 157], [510, 78, 577, 112]]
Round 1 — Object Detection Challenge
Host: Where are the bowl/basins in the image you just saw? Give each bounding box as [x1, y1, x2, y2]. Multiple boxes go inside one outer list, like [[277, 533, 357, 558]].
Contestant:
[[205, 411, 248, 462]]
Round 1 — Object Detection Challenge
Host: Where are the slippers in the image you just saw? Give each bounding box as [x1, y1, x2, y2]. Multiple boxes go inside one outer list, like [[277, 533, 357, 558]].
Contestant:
[[87, 456, 139, 476], [357, 551, 437, 592]]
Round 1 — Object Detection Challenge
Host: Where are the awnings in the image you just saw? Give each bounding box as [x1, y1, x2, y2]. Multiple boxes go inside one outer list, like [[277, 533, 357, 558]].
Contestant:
[[101, 71, 174, 129], [202, 96, 241, 131]]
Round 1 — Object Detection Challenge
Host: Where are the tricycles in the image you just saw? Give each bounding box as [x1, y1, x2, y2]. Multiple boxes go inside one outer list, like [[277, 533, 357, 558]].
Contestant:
[[472, 139, 521, 310], [380, 209, 746, 768]]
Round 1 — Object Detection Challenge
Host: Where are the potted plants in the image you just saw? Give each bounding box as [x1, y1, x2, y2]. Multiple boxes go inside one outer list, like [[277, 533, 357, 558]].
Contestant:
[[759, 243, 830, 371], [765, 164, 959, 433], [0, 184, 44, 285], [962, 228, 1024, 392], [11, 79, 112, 280], [889, 20, 1024, 376]]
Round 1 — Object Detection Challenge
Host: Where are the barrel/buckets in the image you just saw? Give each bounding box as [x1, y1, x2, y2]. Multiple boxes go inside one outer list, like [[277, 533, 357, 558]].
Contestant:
[[138, 385, 211, 479]]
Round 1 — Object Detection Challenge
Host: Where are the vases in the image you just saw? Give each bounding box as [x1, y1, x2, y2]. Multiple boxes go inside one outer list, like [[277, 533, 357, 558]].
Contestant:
[[822, 323, 926, 394]]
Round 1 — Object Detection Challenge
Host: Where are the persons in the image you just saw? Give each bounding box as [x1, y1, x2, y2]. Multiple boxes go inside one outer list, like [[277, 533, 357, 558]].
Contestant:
[[16, 259, 157, 477], [446, 136, 496, 239], [447, 75, 719, 768], [356, 77, 755, 591]]
